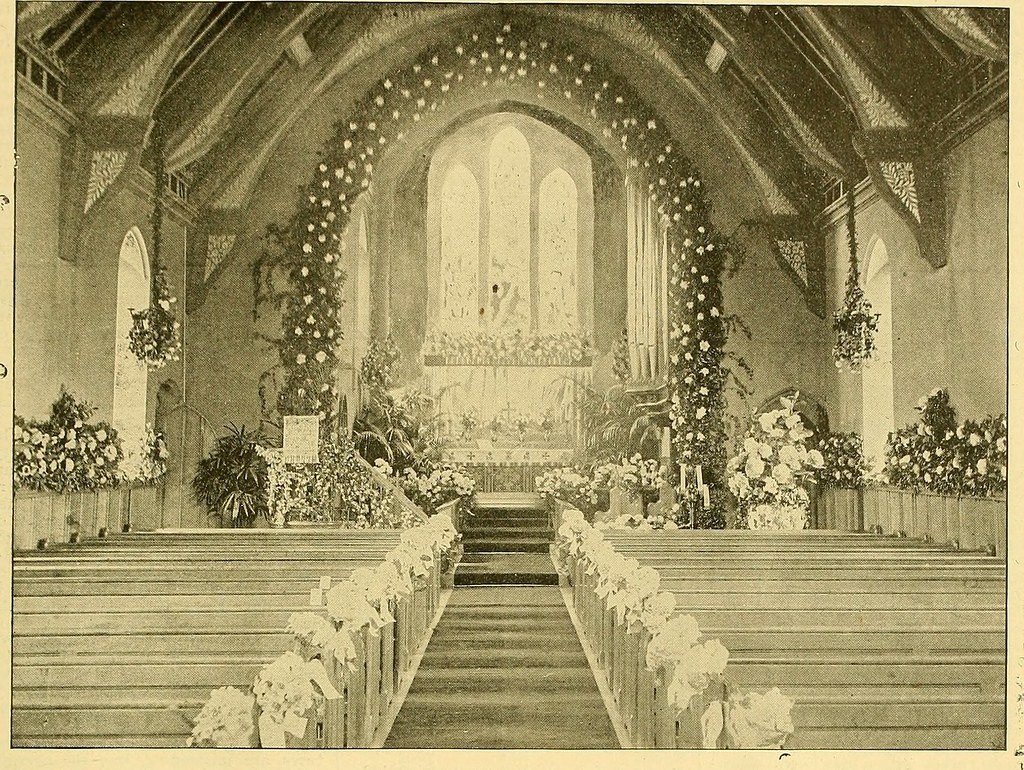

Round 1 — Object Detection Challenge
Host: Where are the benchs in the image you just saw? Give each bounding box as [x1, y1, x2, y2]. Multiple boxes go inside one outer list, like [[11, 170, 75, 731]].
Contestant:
[[573, 536, 1005, 750], [13, 534, 439, 747]]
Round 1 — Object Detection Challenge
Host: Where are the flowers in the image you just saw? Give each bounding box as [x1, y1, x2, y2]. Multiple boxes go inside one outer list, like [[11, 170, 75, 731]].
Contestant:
[[14, 26, 1007, 531], [186, 531, 455, 748], [557, 531, 795, 749]]
